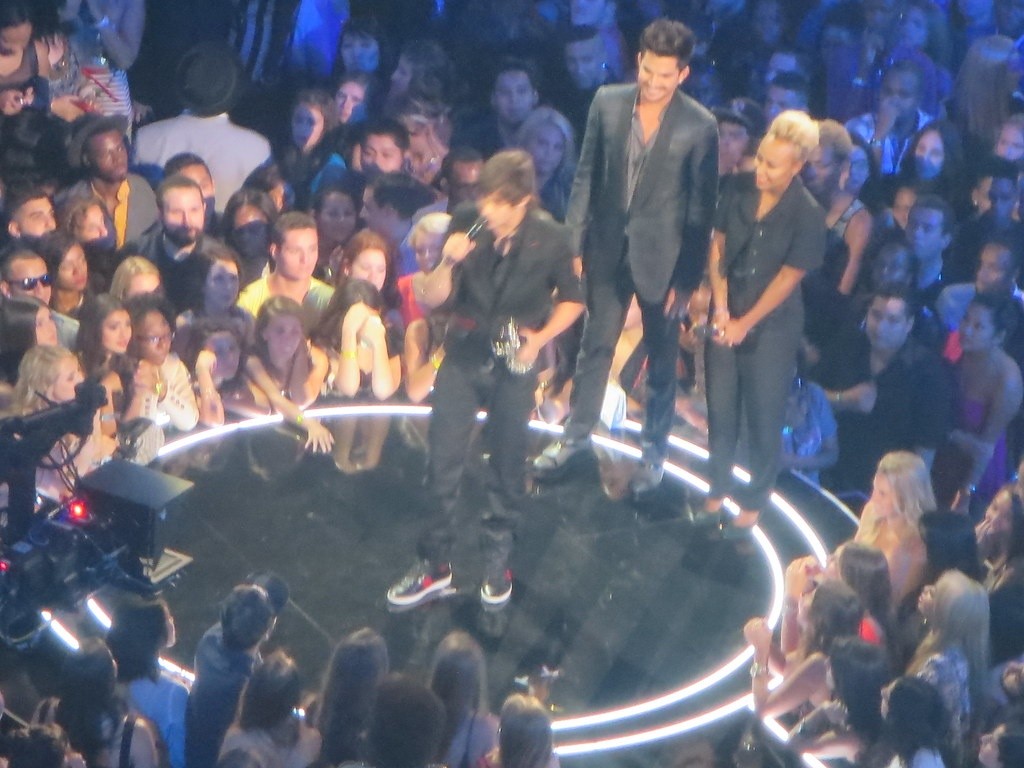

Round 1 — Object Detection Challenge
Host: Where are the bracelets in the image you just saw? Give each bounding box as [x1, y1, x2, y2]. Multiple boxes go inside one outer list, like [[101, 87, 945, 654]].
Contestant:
[[959, 483, 976, 496], [750, 665, 767, 676], [869, 138, 885, 147], [94, 15, 110, 30], [155, 382, 162, 394], [340, 350, 358, 359], [432, 355, 440, 369], [835, 391, 841, 401], [293, 413, 304, 426], [799, 719, 808, 735]]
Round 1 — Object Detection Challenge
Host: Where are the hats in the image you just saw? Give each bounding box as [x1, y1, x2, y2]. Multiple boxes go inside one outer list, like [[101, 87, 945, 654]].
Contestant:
[[172, 40, 246, 117], [707, 95, 765, 136], [218, 568, 290, 650], [65, 108, 132, 177]]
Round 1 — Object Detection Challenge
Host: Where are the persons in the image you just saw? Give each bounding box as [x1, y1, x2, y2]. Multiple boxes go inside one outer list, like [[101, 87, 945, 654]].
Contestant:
[[533, 17, 719, 501], [697, 110, 827, 534], [386, 150, 586, 605], [0, 0, 1024, 768]]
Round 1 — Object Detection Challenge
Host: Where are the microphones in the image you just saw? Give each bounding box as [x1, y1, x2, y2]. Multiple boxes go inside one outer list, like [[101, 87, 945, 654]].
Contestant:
[[696, 324, 722, 337], [445, 215, 488, 268]]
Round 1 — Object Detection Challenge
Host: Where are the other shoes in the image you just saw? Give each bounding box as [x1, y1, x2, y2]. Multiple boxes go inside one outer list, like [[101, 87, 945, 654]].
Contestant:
[[693, 502, 721, 529], [724, 510, 764, 540]]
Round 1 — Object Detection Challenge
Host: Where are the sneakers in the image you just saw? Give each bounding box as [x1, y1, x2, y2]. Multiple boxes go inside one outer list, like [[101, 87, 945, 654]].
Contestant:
[[480, 560, 514, 605], [388, 557, 453, 606]]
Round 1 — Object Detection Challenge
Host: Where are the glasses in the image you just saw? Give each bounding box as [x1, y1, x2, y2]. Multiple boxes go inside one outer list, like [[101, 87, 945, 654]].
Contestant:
[[10, 273, 53, 292], [880, 86, 919, 102], [138, 330, 175, 345]]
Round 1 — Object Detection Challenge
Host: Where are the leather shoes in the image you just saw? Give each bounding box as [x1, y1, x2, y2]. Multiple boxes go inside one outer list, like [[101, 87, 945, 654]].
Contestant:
[[533, 434, 591, 474], [629, 451, 671, 496]]
